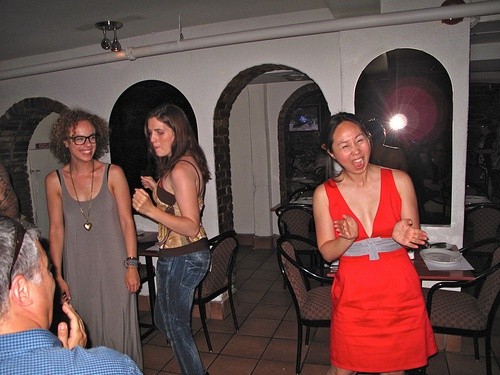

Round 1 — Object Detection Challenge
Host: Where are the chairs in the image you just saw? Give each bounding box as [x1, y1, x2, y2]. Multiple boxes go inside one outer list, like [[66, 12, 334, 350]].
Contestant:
[[463, 204, 500, 278], [276, 206, 324, 290], [165, 235, 240, 353], [409, 237, 500, 375], [275, 234, 335, 375]]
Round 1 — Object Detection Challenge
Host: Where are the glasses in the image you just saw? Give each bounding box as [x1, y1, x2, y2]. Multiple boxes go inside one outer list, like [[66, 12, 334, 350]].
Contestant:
[[64, 134, 99, 145], [0, 215, 27, 289]]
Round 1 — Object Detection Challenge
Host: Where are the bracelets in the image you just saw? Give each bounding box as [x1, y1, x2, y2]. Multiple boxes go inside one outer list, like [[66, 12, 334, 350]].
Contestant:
[[124, 256, 138, 268]]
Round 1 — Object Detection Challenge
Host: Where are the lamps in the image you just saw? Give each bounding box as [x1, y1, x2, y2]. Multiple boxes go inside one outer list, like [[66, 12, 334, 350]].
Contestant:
[[95, 20, 123, 53]]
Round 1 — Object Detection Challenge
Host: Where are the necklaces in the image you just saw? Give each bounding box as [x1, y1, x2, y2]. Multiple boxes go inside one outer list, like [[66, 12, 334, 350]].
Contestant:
[[69, 159, 95, 231]]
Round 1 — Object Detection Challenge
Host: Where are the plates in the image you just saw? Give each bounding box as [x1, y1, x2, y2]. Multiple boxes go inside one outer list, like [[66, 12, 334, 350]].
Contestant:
[[419, 248, 461, 265]]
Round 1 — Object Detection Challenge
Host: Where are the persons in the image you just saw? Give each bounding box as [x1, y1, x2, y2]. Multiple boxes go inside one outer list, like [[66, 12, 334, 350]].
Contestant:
[[132, 105, 210, 375], [0, 163, 143, 375], [313, 112, 438, 375], [45, 106, 144, 371], [365, 117, 427, 215], [467, 118, 500, 201]]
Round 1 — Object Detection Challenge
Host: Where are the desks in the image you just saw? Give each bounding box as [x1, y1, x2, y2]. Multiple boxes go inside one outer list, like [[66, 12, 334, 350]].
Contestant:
[[412, 243, 477, 297], [135, 231, 160, 329]]
